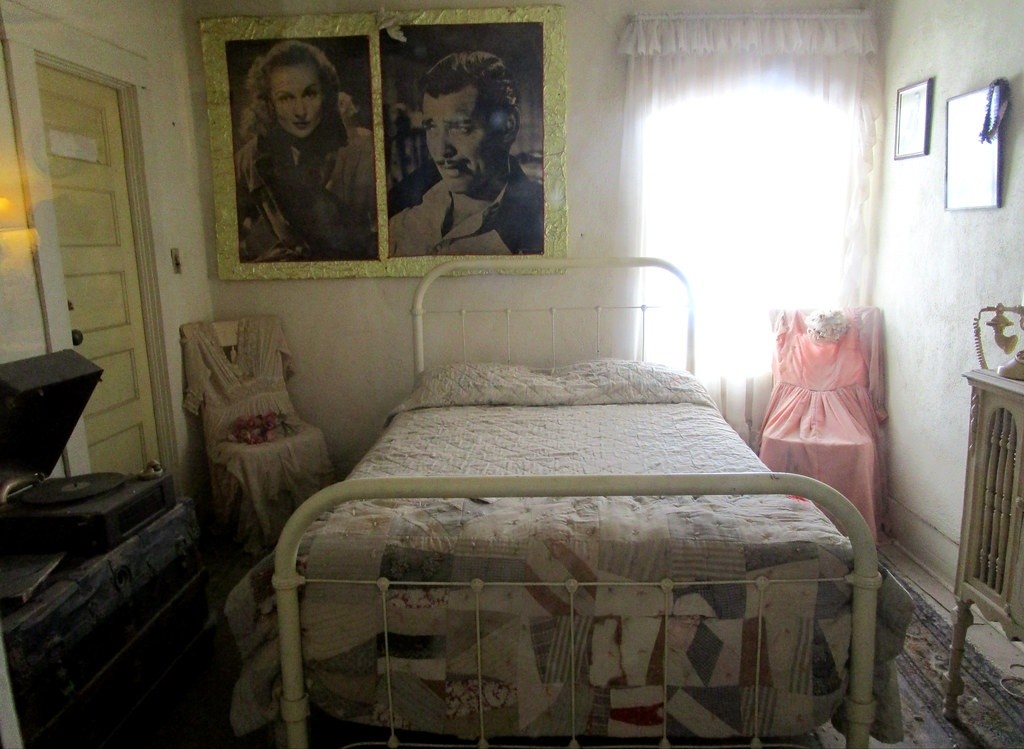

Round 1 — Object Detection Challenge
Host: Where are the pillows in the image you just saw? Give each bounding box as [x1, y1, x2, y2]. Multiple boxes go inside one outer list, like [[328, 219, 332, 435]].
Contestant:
[[379, 359, 720, 429]]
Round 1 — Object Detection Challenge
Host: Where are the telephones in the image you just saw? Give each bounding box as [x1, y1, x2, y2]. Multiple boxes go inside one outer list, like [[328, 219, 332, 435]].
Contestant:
[[985, 304, 1024, 380]]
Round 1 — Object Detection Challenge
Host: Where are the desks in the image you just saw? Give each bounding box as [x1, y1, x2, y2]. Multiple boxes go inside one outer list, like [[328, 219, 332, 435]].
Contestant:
[[942, 369, 1024, 720]]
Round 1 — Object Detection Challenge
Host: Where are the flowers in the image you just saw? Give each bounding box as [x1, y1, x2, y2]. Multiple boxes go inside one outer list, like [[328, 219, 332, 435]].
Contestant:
[[228, 409, 302, 445], [806, 311, 851, 343]]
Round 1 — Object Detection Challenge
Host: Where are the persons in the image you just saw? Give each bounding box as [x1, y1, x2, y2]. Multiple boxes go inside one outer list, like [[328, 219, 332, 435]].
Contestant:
[[234, 40, 377, 262], [388, 53, 543, 256]]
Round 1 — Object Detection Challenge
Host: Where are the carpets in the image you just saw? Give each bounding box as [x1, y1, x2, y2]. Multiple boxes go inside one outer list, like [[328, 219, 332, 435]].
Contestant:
[[812, 552, 1024, 749]]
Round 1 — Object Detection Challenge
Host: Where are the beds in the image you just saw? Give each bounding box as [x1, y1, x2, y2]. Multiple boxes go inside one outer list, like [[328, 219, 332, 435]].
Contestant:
[[219, 257, 916, 749]]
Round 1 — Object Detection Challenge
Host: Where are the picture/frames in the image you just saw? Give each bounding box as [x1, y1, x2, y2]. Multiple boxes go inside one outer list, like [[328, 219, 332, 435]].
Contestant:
[[943, 84, 1002, 210], [893, 78, 934, 159], [198, 4, 570, 280]]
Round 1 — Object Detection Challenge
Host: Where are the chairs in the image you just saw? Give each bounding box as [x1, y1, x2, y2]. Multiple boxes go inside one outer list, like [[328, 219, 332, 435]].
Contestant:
[[753, 306, 894, 551], [179, 315, 334, 558]]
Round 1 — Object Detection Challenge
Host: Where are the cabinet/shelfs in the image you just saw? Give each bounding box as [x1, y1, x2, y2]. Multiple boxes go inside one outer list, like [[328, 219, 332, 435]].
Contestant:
[[0, 497, 221, 749]]
